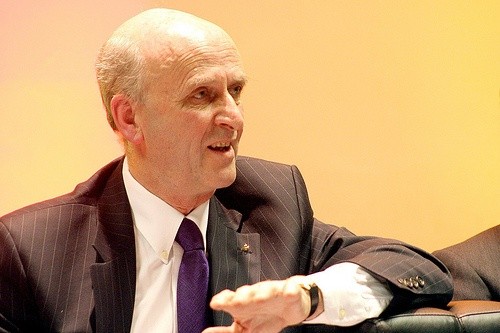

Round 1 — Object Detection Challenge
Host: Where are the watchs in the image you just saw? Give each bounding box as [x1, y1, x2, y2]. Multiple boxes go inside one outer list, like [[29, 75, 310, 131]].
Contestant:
[[286, 275, 321, 322]]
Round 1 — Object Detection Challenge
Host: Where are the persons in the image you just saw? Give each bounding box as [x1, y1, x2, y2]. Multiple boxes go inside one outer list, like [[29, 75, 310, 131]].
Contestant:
[[0, 7, 453, 332]]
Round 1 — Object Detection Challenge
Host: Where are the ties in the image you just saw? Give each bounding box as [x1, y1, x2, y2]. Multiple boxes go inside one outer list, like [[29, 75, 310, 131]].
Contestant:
[[174, 218, 214, 333]]
